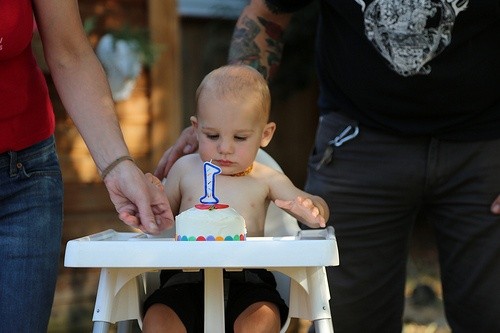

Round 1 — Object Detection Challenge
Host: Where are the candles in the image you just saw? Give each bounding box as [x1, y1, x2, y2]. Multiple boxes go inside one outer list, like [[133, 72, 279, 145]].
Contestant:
[[199, 158, 222, 205]]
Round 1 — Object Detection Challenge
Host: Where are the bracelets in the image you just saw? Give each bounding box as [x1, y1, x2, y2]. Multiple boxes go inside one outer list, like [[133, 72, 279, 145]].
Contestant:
[[100, 155, 135, 183]]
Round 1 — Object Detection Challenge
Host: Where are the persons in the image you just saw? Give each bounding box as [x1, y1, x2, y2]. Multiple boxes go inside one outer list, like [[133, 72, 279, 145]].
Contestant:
[[83, 0, 149, 103], [139, 63, 330, 333], [149, 0, 500, 333], [0, 0, 177, 333]]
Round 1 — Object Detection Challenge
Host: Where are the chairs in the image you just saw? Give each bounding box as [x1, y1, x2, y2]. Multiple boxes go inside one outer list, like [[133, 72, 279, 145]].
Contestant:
[[92, 148, 335, 333]]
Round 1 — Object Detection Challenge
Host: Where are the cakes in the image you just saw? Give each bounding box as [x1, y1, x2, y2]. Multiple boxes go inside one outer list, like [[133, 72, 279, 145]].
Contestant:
[[175, 204, 247, 241]]
